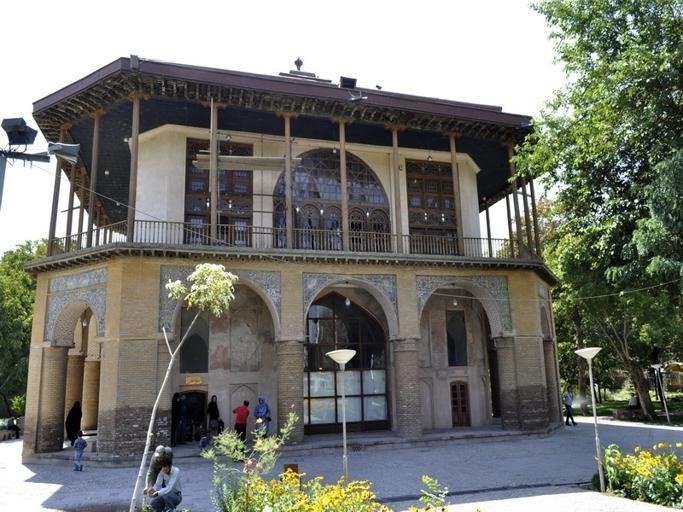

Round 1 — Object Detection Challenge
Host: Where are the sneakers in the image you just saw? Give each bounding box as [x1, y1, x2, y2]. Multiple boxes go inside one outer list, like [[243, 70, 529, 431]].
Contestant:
[[73, 465, 83, 471], [566, 423, 578, 426]]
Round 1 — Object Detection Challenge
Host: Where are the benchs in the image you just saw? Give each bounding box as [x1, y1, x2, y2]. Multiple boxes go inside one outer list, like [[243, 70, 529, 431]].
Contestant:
[[86, 437, 97, 451], [0, 430, 12, 441], [613, 409, 641, 420]]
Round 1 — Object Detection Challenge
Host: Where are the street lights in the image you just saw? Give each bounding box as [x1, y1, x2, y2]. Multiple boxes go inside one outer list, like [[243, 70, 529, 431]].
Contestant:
[[651, 363, 671, 424], [325, 349, 359, 485], [573, 345, 606, 493]]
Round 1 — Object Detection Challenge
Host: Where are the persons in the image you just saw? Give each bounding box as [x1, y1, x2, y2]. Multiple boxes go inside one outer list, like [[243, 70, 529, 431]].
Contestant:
[[73, 431, 88, 471], [207, 395, 219, 441], [142, 445, 182, 512], [66, 401, 82, 446], [562, 386, 578, 426], [232, 400, 249, 452], [7, 416, 21, 439], [253, 396, 271, 438], [626, 393, 637, 409]]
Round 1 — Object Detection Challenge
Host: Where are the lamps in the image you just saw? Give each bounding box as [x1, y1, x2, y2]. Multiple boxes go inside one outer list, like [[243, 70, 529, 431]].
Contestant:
[[451, 283, 457, 306], [82, 310, 87, 329], [345, 281, 350, 305]]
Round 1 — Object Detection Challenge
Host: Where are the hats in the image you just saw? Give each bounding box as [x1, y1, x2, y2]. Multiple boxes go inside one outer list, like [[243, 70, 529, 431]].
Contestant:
[[154, 445, 165, 457]]
